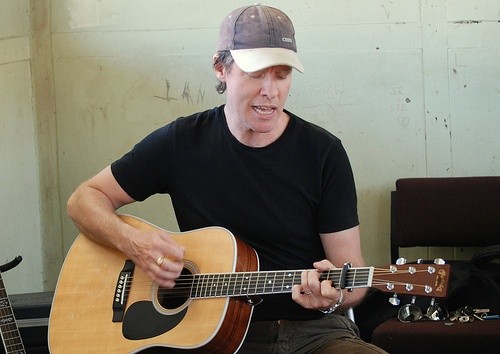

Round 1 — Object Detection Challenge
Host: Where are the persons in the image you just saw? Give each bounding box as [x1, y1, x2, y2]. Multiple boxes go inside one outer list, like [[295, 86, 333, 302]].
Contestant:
[[48, 2, 450, 354]]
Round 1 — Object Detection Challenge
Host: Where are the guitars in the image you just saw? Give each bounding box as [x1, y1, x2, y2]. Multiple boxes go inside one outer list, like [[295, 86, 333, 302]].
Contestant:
[[0, 271, 27, 354], [48, 213, 451, 354]]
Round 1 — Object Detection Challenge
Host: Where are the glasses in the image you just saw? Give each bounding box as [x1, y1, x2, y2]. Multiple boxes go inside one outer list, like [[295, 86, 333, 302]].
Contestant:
[[396, 302, 449, 324]]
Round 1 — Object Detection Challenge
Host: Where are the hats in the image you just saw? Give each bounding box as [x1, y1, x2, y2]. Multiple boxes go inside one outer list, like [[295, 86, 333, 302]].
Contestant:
[[216, 3, 304, 74]]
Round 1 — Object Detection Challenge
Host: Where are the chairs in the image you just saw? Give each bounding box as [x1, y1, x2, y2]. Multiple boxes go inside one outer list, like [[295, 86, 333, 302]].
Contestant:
[[351, 176, 500, 354]]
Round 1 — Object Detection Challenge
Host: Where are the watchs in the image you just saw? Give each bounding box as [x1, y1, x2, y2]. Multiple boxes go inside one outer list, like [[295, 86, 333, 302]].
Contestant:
[[318, 289, 343, 313]]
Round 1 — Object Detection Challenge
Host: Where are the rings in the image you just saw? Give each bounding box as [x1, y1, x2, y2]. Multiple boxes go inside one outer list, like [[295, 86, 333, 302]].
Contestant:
[[300, 289, 312, 296], [157, 255, 165, 266]]
